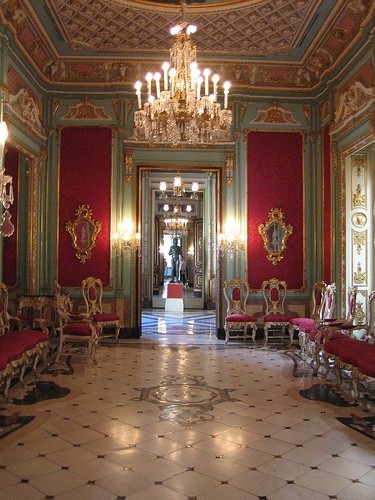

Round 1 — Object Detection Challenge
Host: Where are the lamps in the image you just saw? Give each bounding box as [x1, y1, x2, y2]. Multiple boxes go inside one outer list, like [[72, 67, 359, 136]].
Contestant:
[[0, 119, 14, 209], [112, 220, 142, 259], [217, 221, 246, 255], [164, 217, 187, 231], [133, 0, 233, 145], [162, 200, 192, 216], [159, 171, 199, 199]]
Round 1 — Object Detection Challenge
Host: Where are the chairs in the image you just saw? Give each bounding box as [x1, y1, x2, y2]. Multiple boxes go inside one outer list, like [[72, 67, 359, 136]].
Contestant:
[[261, 277, 375, 413], [223, 277, 258, 345], [0, 277, 121, 401]]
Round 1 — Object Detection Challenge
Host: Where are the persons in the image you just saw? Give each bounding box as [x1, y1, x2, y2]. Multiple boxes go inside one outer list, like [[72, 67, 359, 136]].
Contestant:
[[168, 238, 184, 283], [179, 254, 186, 287]]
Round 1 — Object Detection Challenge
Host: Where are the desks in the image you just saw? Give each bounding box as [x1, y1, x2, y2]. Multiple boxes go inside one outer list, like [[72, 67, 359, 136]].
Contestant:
[[16, 289, 69, 331]]
